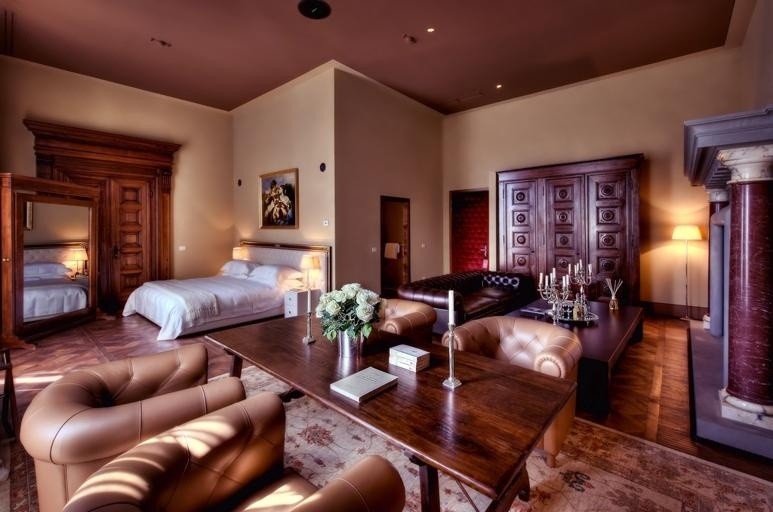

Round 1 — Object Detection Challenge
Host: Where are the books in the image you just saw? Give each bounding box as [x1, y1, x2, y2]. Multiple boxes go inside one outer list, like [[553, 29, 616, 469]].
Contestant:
[[330, 367, 399, 404]]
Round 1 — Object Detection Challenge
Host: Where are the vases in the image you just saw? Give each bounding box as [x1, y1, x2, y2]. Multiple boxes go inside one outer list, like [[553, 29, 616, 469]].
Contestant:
[[341, 329, 356, 376]]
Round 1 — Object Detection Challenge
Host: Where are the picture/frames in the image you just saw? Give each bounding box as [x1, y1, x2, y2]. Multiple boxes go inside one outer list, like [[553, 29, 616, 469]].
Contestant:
[[258, 168, 299, 229]]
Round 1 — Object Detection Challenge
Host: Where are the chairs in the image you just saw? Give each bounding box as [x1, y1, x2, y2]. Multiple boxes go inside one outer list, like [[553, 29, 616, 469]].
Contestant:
[[376, 299, 437, 346], [64, 391, 406, 512], [442, 316, 583, 467], [18, 344, 246, 512]]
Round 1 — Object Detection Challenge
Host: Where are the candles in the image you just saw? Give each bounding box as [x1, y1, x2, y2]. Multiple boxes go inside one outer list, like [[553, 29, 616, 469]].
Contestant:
[[536, 259, 592, 317]]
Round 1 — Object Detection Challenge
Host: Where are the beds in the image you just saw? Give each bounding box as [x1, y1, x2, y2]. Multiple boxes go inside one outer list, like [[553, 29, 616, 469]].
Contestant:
[[122, 243, 326, 341], [23, 244, 87, 322]]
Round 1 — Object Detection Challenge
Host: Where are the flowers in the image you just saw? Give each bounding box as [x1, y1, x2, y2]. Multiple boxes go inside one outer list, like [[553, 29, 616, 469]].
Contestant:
[[315, 282, 381, 341]]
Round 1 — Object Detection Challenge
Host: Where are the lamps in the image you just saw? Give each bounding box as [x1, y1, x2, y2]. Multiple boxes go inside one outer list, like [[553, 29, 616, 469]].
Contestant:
[[672, 225, 703, 319], [67, 249, 89, 278], [299, 255, 321, 291]]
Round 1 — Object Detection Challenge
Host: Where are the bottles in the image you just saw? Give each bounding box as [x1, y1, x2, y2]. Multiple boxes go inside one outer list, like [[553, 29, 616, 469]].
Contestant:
[[608, 295, 618, 311], [562, 298, 588, 320]]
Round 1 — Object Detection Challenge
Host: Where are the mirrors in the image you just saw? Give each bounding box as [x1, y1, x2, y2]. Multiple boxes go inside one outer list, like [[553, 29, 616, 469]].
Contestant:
[[15, 192, 95, 333]]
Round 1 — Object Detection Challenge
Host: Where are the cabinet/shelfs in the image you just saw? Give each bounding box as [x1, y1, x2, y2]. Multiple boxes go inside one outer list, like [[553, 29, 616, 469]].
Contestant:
[[496, 152, 640, 306]]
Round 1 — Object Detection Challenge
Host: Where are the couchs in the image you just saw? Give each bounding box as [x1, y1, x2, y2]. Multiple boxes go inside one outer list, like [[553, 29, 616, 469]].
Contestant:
[[397, 270, 532, 334]]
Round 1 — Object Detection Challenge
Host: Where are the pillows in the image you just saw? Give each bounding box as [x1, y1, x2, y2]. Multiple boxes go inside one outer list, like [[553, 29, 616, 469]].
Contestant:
[[23, 262, 70, 281], [220, 259, 304, 290]]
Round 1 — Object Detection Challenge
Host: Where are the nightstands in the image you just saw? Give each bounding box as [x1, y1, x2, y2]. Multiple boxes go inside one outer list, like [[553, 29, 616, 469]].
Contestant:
[[284, 289, 319, 317]]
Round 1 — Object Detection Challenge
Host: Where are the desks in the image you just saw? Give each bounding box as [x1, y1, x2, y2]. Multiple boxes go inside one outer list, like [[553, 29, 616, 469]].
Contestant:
[[205, 318, 577, 512], [501, 298, 643, 416]]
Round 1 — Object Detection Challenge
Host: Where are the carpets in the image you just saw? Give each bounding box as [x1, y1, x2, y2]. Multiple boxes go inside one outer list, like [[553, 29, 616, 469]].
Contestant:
[[206, 365, 682, 512]]
